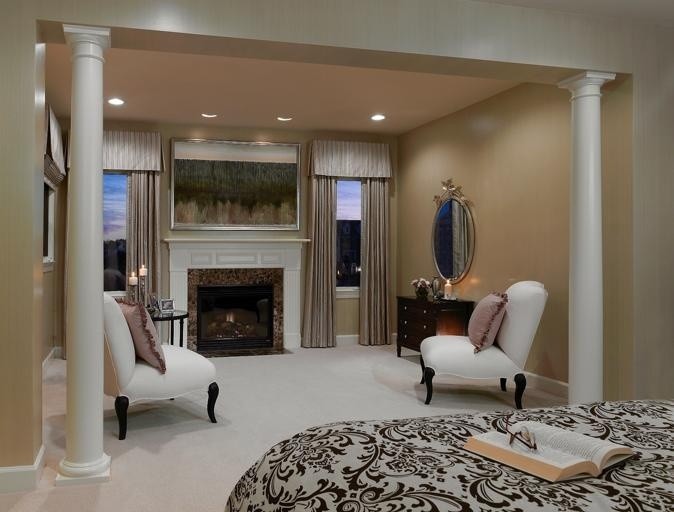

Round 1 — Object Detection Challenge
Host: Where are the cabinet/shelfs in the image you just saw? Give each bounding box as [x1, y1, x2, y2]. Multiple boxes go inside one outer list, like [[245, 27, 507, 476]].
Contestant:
[[395, 295, 475, 358]]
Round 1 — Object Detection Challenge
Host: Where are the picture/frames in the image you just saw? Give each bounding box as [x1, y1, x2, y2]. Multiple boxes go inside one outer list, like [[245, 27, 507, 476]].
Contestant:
[[169, 137, 302, 232]]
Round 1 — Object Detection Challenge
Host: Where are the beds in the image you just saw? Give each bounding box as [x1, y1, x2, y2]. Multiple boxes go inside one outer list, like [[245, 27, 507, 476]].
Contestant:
[[255, 400, 674, 511]]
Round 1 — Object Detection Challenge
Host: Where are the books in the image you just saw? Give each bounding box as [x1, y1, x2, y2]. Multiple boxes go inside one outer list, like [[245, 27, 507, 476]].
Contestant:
[[463, 419, 636, 483]]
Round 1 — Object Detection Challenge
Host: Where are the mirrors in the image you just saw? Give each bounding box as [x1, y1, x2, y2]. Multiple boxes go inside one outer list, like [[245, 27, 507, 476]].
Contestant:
[[429, 179, 478, 285]]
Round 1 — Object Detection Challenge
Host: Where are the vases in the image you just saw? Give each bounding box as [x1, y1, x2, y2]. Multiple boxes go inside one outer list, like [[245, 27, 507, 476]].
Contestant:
[[432, 276, 441, 295], [416, 288, 428, 298]]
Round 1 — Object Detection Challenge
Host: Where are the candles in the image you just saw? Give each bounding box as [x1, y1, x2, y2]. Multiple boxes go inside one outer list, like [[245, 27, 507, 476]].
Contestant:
[[139, 264, 147, 276], [128, 272, 138, 285], [444, 279, 452, 297]]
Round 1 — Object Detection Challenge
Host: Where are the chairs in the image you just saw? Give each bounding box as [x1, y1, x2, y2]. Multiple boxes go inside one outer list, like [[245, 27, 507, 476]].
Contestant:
[[418, 280, 548, 409], [102, 294, 219, 441]]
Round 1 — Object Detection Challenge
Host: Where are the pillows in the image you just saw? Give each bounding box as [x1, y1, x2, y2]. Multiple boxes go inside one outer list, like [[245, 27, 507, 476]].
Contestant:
[[113, 296, 166, 375], [468, 291, 509, 354]]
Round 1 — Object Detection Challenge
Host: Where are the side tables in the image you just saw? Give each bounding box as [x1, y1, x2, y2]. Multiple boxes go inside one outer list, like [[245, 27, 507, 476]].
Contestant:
[[149, 307, 189, 347]]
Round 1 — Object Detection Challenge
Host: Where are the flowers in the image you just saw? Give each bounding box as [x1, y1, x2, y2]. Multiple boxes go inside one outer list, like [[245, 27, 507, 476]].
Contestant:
[[410, 277, 432, 289]]
